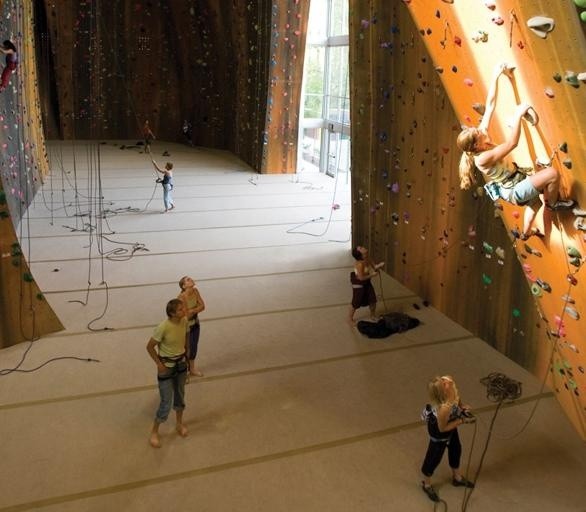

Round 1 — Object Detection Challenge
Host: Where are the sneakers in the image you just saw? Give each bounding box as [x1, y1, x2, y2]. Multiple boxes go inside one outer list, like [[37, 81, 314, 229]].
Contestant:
[[422, 480, 440, 503], [545, 199, 578, 211], [452, 476, 475, 489], [521, 227, 540, 241]]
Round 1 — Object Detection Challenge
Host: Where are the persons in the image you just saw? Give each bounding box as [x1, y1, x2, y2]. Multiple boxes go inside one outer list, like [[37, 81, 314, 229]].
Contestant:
[[0, 39, 19, 93], [348, 246, 380, 327], [177, 274, 203, 377], [455, 64, 579, 241], [142, 118, 157, 153], [421, 375, 474, 501], [152, 159, 176, 212], [145, 298, 189, 449]]
